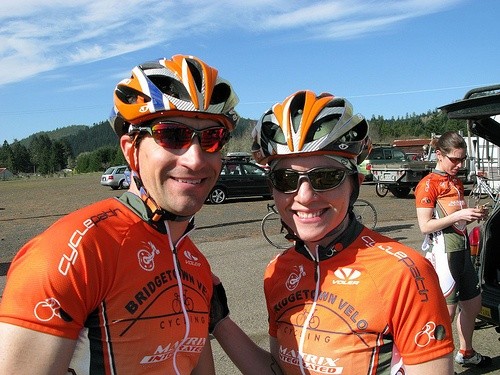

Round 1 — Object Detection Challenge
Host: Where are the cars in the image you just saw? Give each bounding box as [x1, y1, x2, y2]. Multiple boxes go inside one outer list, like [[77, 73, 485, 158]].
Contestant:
[[100, 165, 131, 190], [458, 205, 500, 329], [204, 151, 273, 205]]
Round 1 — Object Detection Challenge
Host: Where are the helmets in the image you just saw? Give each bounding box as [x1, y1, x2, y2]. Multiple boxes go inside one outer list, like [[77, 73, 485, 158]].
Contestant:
[[250, 90, 369, 164], [108, 53, 239, 139]]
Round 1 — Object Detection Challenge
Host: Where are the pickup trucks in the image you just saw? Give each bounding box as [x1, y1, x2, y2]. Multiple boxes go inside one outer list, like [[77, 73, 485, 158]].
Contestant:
[[357, 143, 475, 199]]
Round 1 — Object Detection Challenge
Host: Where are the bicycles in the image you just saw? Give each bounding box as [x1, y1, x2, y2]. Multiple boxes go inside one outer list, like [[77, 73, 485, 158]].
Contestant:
[[467, 173, 500, 209], [260, 197, 378, 250]]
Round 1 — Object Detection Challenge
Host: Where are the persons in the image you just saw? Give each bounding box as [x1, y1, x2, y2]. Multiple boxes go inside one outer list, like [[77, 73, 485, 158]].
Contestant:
[[0, 54, 239, 375], [415, 132, 492, 366], [209, 90, 454, 375]]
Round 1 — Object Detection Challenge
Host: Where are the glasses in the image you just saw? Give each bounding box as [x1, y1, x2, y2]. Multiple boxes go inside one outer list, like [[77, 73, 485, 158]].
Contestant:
[[128, 121, 231, 154], [266, 166, 358, 194], [443, 154, 467, 164]]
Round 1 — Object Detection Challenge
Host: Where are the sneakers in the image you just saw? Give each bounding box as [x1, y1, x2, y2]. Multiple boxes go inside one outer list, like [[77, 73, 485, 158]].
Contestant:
[[455, 349, 491, 367]]
